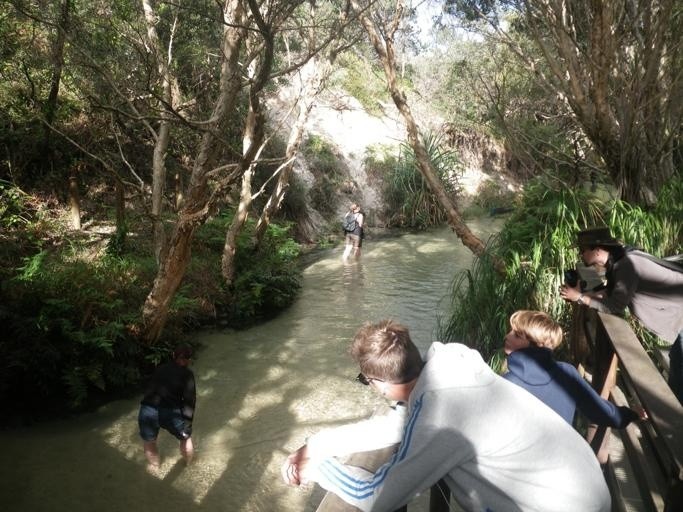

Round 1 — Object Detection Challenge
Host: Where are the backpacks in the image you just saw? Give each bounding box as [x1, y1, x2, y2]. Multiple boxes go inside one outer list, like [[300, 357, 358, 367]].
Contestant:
[[341, 211, 360, 232]]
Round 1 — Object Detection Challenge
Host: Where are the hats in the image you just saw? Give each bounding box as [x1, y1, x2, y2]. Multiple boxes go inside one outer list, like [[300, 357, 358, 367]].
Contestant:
[[565, 225, 625, 249]]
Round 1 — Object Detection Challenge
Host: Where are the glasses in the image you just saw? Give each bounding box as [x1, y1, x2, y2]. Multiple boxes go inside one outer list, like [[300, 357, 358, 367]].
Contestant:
[[354, 373, 385, 387]]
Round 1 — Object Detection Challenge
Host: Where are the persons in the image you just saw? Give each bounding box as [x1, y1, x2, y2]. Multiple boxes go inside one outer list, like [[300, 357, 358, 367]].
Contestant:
[[493, 307, 649, 438], [342, 204, 364, 261], [554, 222, 682, 407], [350, 205, 365, 256], [137, 344, 196, 472], [278, 316, 613, 512]]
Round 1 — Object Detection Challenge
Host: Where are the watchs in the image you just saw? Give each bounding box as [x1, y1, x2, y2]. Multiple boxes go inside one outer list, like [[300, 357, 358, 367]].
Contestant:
[[576, 293, 585, 305]]
